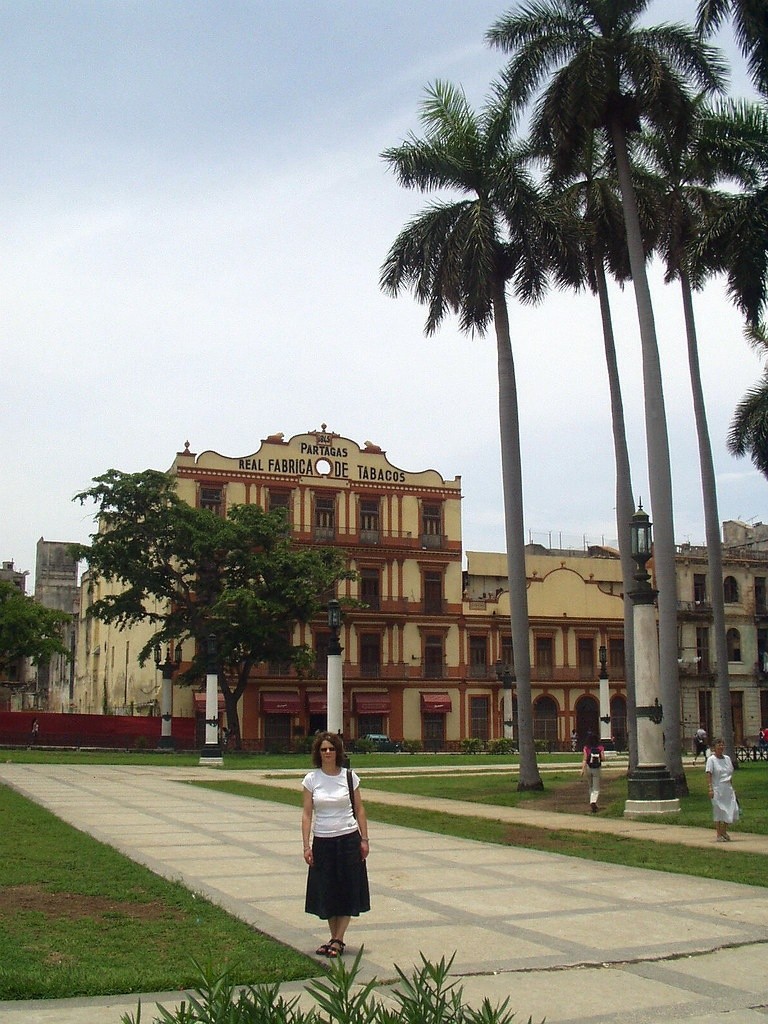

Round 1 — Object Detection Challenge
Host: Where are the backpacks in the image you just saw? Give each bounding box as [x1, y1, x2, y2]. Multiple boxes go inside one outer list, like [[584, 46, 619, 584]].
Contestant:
[[586, 745, 601, 768], [694, 733, 704, 746]]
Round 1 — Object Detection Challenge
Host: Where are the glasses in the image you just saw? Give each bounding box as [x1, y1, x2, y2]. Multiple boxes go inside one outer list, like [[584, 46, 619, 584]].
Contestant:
[[320, 747, 335, 752]]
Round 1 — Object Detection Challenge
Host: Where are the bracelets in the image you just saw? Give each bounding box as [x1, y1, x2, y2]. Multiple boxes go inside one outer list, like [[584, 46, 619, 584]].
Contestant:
[[707, 783, 713, 792], [304, 846, 310, 852], [362, 837, 370, 841]]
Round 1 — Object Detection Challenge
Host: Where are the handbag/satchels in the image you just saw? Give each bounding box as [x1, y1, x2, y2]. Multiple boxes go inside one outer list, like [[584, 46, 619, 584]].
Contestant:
[[705, 747, 711, 757]]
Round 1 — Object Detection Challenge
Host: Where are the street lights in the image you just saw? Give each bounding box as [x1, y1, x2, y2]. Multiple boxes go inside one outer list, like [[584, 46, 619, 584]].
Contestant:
[[624, 495, 684, 817], [322, 598, 343, 739], [152, 641, 183, 750], [198, 631, 225, 766], [502, 667, 513, 742], [598, 644, 616, 754]]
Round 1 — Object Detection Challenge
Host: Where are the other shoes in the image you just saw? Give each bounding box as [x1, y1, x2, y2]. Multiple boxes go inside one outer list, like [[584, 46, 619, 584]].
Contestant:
[[717, 836, 726, 842], [591, 804, 597, 812], [721, 833, 730, 840]]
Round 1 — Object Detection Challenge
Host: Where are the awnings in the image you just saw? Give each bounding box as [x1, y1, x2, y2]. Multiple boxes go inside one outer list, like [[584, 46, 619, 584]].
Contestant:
[[421, 692, 452, 713], [306, 691, 351, 715], [192, 689, 229, 713], [354, 691, 393, 715], [258, 690, 302, 715]]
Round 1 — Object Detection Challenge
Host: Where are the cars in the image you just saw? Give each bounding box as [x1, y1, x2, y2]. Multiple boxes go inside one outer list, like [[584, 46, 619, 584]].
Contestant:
[[365, 733, 391, 746]]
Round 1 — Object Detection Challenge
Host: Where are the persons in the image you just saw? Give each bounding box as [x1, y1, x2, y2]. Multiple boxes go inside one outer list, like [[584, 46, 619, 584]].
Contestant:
[[30, 718, 39, 744], [302, 732, 370, 957], [693, 724, 709, 764], [703, 736, 739, 840], [757, 726, 768, 750], [221, 725, 233, 755], [580, 731, 605, 809]]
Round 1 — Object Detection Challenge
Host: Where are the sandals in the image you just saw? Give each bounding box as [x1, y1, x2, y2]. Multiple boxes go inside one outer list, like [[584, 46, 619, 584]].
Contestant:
[[326, 940, 345, 958], [316, 939, 334, 955]]
